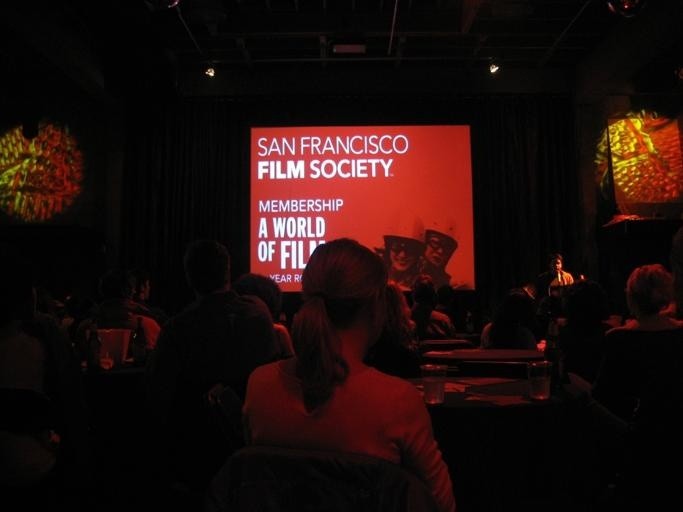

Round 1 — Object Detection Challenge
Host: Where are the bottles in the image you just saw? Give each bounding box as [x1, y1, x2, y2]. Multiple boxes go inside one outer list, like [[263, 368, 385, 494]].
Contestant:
[[132, 316, 148, 367]]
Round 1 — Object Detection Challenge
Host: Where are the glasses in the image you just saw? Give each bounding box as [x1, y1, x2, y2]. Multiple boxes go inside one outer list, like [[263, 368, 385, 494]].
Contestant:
[[389, 238, 420, 255], [424, 233, 455, 254]]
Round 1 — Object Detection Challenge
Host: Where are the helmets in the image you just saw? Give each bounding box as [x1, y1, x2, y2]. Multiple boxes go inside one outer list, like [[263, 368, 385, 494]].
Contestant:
[[381, 220, 426, 247], [422, 217, 460, 250]]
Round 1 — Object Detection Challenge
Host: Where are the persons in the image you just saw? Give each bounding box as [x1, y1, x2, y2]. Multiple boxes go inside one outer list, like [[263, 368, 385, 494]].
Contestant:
[[417, 229, 458, 289], [0, 239, 683, 512], [379, 234, 424, 292]]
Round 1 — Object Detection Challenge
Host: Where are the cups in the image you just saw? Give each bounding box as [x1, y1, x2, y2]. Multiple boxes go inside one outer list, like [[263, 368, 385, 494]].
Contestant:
[[526, 361, 551, 401], [419, 364, 448, 405]]
